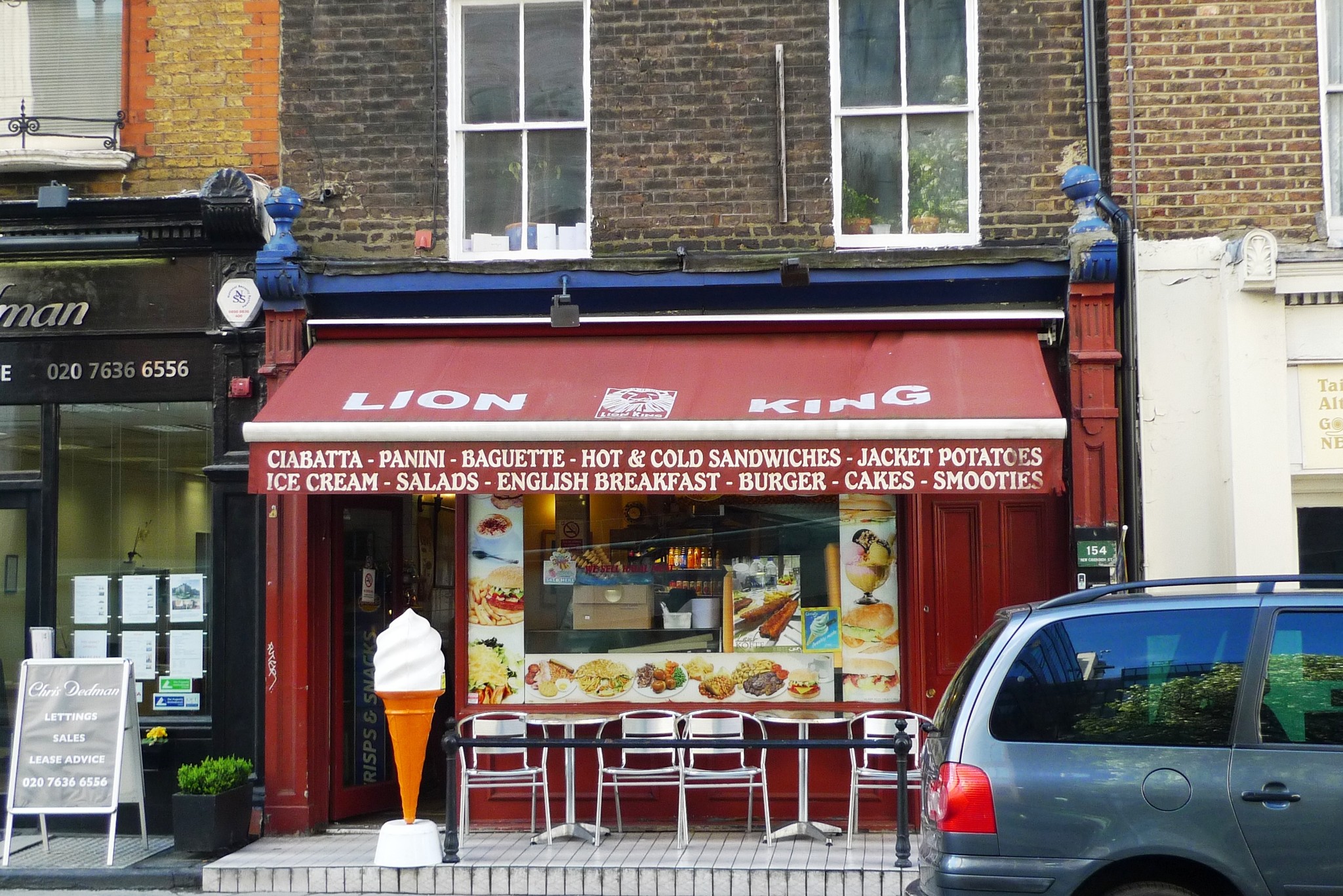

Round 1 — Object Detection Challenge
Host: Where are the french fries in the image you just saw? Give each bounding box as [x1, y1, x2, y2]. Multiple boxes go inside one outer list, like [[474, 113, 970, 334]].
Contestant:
[[469, 576, 523, 625]]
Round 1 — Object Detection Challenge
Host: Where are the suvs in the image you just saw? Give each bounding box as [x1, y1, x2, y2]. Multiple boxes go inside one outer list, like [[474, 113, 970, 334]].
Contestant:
[[904, 574, 1343, 896]]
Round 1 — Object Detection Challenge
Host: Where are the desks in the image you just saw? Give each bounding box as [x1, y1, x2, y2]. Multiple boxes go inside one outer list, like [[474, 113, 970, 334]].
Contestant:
[[753, 708, 857, 847], [519, 711, 623, 846]]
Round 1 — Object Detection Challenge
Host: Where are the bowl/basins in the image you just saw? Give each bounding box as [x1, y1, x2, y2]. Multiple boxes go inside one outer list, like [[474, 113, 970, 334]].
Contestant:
[[776, 584, 795, 593], [663, 612, 692, 628]]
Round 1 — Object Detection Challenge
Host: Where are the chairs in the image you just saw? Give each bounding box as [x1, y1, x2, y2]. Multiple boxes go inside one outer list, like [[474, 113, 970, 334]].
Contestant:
[[845, 709, 933, 850], [674, 708, 773, 850], [456, 710, 553, 849], [594, 707, 689, 851]]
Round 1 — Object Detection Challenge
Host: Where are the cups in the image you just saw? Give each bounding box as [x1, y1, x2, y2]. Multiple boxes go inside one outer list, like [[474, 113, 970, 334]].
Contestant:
[[808, 656, 831, 678], [474, 512, 513, 555], [845, 564, 890, 605], [733, 563, 750, 583]]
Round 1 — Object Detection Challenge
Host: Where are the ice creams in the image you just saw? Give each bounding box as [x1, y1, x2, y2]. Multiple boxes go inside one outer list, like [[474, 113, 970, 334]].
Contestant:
[[807, 611, 834, 645]]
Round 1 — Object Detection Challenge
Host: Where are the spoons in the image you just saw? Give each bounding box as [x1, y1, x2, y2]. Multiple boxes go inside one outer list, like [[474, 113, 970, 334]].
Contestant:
[[472, 550, 519, 563]]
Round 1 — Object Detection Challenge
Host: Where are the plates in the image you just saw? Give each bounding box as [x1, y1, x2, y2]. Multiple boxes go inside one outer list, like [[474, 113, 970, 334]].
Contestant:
[[735, 678, 789, 699], [633, 663, 688, 698], [524, 681, 577, 700], [575, 660, 634, 699], [817, 670, 834, 683]]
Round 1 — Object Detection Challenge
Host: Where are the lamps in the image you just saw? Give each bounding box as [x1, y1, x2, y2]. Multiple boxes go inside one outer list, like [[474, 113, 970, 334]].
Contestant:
[[780, 257, 812, 288], [37, 179, 70, 210], [549, 273, 580, 327]]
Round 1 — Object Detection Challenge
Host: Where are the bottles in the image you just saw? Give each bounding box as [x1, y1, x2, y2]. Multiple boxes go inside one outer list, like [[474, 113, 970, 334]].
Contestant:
[[750, 556, 765, 599], [663, 546, 723, 595], [765, 558, 778, 593]]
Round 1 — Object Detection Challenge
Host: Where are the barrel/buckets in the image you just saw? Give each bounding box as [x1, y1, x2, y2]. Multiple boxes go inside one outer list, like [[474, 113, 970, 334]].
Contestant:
[[690, 597, 719, 628]]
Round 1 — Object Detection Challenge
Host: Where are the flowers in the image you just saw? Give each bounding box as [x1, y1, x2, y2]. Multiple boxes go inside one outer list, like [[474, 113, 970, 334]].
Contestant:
[[141, 725, 170, 745]]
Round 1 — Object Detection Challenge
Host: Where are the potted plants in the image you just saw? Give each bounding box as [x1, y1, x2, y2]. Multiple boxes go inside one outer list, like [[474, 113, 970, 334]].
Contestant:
[[908, 147, 941, 234], [172, 754, 255, 851], [841, 179, 880, 235], [868, 213, 893, 234]]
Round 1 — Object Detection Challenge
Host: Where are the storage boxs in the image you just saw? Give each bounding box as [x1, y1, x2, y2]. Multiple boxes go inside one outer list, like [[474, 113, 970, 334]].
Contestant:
[[572, 584, 654, 630]]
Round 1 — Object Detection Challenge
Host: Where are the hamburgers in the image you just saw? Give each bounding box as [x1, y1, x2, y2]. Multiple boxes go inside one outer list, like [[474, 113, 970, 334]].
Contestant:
[[486, 565, 524, 615], [839, 601, 900, 701], [787, 665, 821, 699]]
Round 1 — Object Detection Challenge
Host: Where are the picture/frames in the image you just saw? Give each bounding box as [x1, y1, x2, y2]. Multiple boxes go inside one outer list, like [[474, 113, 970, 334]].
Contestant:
[[432, 504, 455, 589], [538, 529, 558, 609]]
